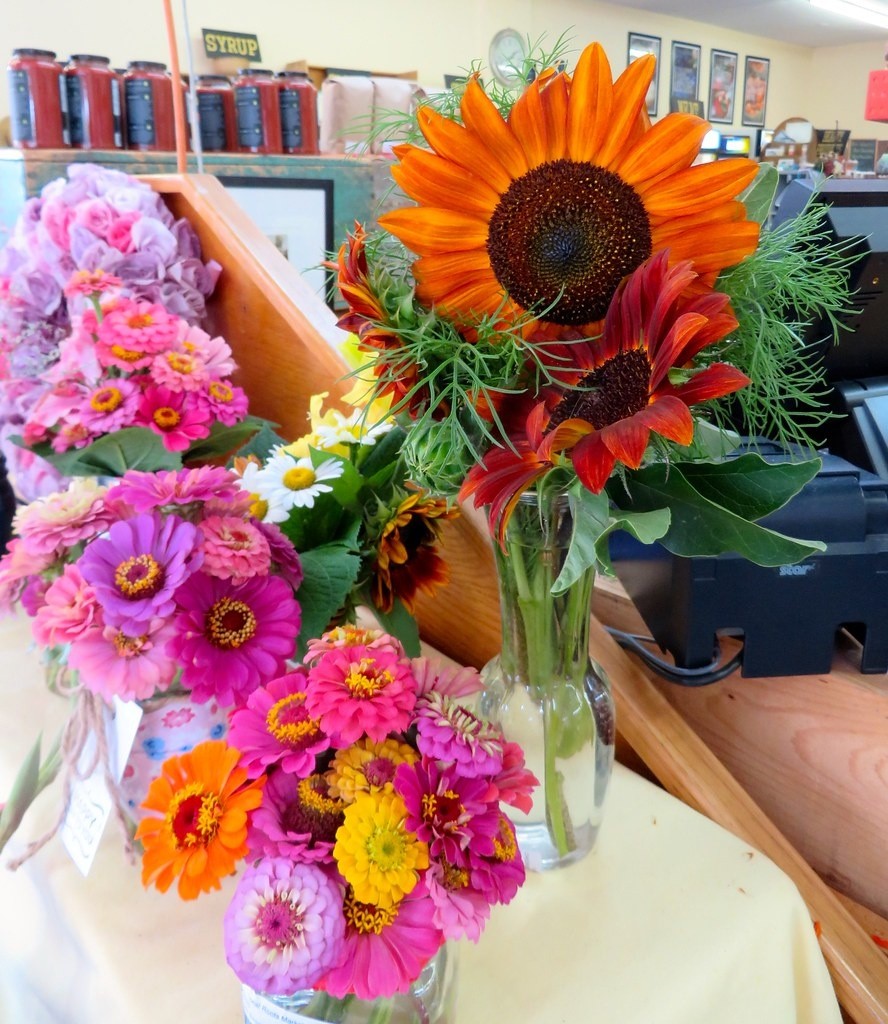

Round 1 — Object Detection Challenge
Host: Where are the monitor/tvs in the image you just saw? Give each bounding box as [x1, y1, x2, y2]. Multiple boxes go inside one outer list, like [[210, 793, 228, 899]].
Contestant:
[[704, 176, 888, 475]]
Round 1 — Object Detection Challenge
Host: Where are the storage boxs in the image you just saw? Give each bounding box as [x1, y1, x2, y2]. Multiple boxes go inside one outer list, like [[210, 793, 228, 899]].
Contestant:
[[760, 141, 813, 170]]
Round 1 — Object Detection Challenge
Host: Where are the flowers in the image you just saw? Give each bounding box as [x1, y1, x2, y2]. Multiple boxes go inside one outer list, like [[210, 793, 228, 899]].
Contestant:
[[0, 161, 541, 998], [307, 23, 873, 852]]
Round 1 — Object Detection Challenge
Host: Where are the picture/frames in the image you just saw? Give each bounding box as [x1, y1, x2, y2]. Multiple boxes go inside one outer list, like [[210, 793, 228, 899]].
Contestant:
[[628, 30, 662, 117], [741, 55, 771, 128], [669, 39, 701, 116], [708, 48, 739, 124]]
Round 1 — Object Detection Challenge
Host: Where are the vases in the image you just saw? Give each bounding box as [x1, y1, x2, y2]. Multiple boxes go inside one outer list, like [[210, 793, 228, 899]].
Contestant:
[[473, 490, 617, 869], [243, 936, 457, 1024]]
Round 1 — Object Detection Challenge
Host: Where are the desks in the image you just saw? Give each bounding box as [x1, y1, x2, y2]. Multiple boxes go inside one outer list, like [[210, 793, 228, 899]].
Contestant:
[[0, 147, 411, 324]]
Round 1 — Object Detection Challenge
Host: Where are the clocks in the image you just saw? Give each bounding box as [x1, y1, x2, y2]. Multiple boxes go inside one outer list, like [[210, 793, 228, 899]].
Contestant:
[[487, 27, 529, 87]]
